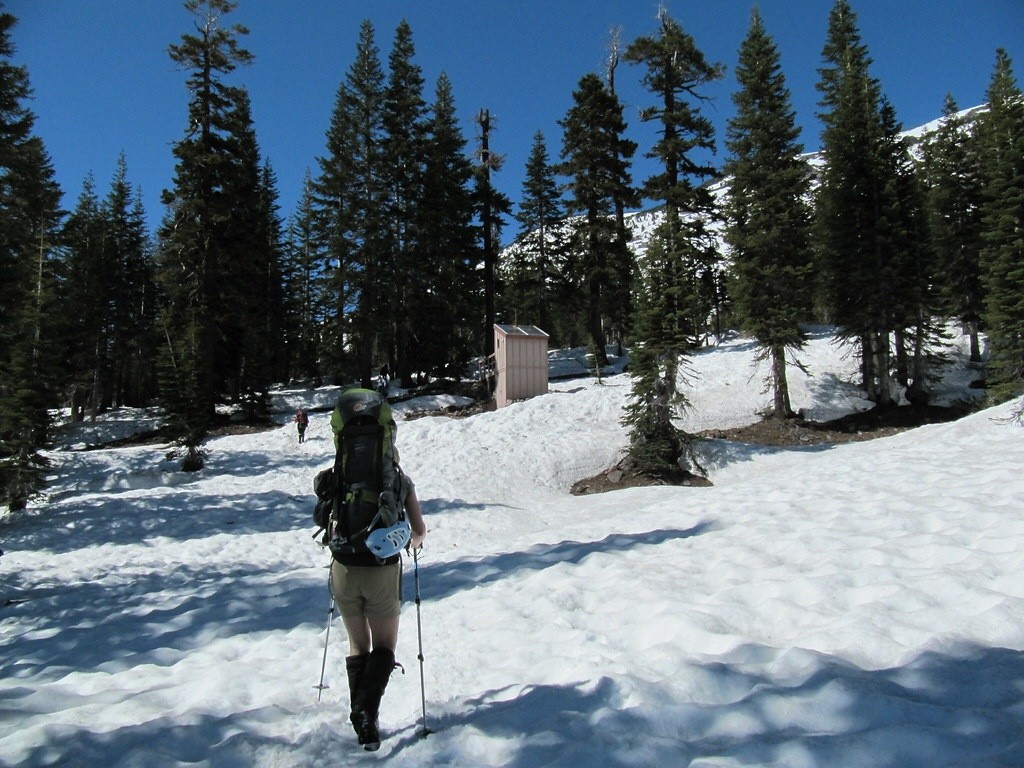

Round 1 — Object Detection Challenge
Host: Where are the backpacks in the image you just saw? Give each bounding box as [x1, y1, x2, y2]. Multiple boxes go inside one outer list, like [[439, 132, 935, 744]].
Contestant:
[[326, 389, 406, 567]]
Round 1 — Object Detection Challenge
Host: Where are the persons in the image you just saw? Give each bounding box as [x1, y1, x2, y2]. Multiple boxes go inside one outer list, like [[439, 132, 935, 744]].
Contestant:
[[327, 446, 426, 751], [294, 411, 307, 444]]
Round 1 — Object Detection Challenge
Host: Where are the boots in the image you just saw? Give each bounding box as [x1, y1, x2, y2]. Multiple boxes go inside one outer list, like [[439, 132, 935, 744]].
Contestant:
[[346, 648, 395, 751]]
